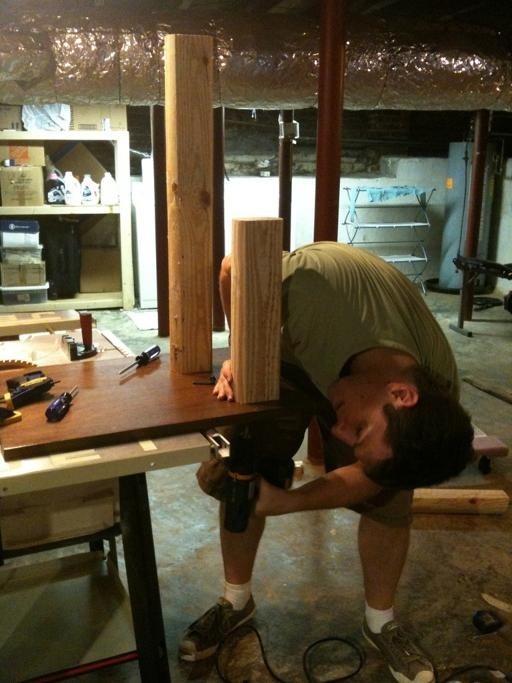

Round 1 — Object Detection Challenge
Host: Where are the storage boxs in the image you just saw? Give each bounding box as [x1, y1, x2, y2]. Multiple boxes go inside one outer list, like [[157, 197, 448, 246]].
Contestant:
[[1, 142, 47, 207], [1, 217, 50, 306], [0, 101, 130, 129]]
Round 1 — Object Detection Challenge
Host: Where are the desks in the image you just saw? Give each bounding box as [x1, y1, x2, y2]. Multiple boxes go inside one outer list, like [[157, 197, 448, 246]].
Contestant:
[[0, 340, 209, 683]]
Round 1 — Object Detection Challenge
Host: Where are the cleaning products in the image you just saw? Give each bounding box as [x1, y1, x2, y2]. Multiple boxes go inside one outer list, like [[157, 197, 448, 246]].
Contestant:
[[44, 171, 119, 206]]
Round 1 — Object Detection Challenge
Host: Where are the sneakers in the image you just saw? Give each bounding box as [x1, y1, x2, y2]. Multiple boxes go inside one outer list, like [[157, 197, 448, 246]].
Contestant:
[[177, 592, 256, 662], [361, 615, 436, 683]]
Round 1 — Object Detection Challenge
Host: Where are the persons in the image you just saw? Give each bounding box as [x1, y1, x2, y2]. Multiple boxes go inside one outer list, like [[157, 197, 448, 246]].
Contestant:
[[178, 240, 474, 683]]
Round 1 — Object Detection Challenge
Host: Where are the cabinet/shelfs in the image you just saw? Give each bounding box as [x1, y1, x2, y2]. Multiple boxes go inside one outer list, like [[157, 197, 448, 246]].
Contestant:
[[0, 127, 136, 314], [343, 181, 437, 297]]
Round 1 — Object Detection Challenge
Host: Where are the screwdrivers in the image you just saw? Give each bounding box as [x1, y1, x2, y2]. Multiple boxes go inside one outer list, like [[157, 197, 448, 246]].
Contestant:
[[118, 344, 161, 376], [45, 385, 79, 421]]
